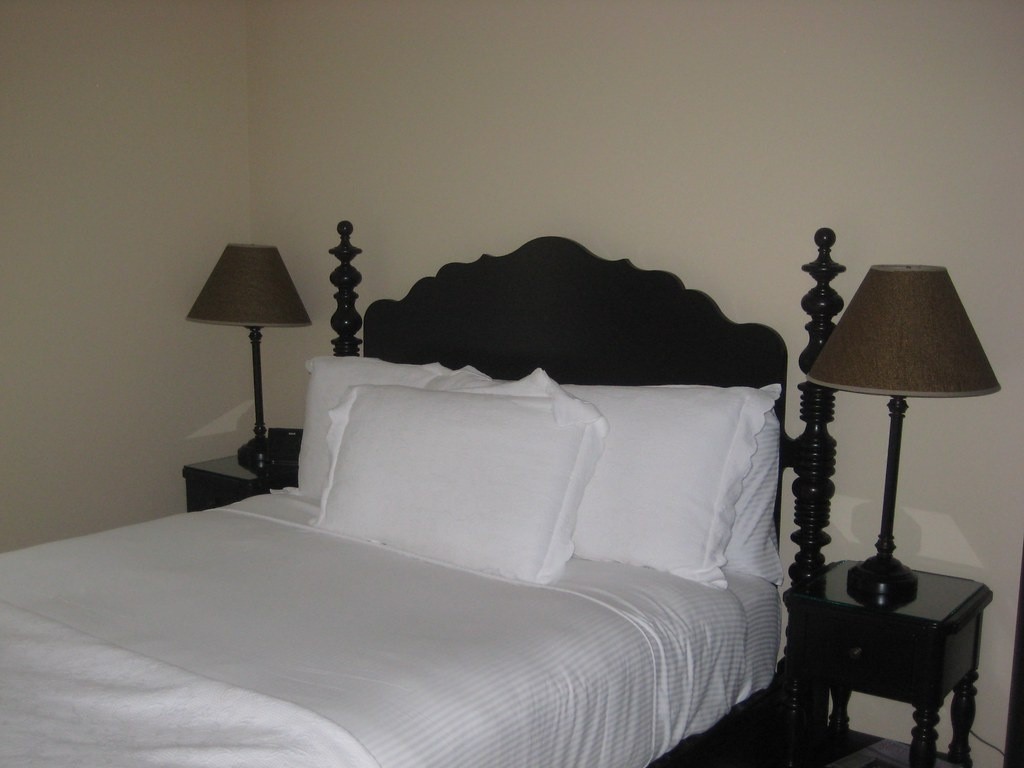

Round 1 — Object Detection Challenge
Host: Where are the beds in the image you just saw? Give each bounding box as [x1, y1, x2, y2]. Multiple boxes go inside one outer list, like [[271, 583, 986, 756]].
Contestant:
[[0, 219, 850, 768]]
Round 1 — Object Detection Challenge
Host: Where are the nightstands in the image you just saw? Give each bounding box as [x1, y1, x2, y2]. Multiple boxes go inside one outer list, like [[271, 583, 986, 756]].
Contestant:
[[180, 454, 264, 513], [776, 559, 995, 768]]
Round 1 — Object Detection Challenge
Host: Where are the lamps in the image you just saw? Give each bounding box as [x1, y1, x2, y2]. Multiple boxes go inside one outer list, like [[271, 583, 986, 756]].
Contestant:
[[808, 264, 1002, 607], [186, 244, 313, 466]]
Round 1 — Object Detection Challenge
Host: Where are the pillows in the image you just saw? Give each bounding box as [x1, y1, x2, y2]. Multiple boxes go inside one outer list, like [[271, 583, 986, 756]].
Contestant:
[[284, 355, 493, 512], [726, 408, 783, 587], [559, 384, 784, 591], [312, 382, 612, 585]]
